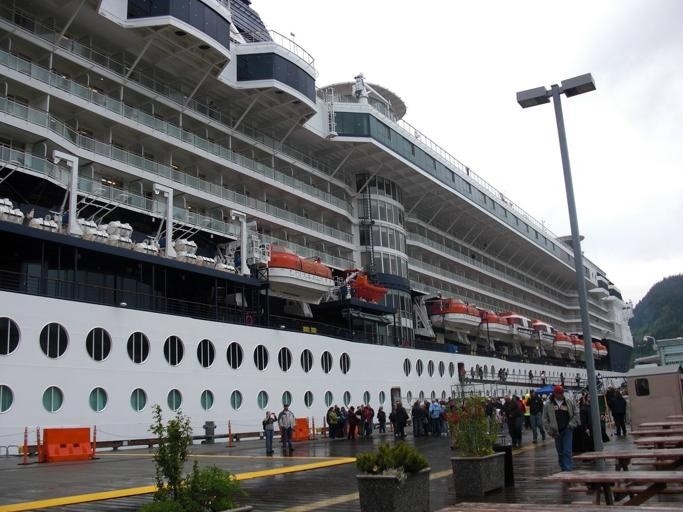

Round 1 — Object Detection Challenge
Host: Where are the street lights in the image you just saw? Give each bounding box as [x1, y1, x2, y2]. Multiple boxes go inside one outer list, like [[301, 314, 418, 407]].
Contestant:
[[151, 182, 177, 259], [228, 208, 250, 276], [508, 67, 608, 472], [640, 333, 656, 351], [51, 149, 84, 238]]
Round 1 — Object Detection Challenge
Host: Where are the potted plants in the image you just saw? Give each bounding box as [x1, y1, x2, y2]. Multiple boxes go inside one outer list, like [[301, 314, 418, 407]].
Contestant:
[[134, 404, 253, 512], [356, 440, 431, 512], [448, 395, 506, 497]]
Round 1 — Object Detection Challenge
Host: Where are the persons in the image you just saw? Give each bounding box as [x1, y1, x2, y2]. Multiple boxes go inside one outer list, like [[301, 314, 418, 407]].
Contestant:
[[262, 410, 277, 454], [543, 385, 581, 472], [277, 404, 297, 452], [325, 366, 628, 450]]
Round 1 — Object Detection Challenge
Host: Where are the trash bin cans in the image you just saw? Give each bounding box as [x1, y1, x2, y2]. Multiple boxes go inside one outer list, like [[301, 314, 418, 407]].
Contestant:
[[572, 404, 595, 453], [493, 434, 515, 488]]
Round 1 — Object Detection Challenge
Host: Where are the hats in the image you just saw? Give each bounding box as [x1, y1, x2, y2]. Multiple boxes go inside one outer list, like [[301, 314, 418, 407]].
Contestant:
[[554, 386, 564, 393]]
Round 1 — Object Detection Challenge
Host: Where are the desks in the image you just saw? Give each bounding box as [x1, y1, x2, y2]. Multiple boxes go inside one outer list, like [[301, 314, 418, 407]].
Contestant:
[[435, 415, 683, 512]]
